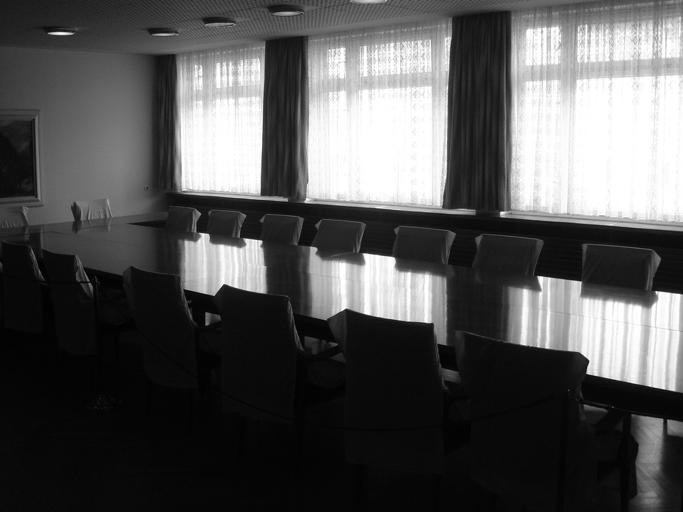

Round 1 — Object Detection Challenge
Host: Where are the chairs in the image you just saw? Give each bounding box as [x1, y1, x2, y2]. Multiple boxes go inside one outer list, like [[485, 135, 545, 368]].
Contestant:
[[580, 241, 663, 290]]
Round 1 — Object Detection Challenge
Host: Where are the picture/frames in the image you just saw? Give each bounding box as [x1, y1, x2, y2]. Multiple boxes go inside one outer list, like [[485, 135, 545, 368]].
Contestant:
[[0, 110, 41, 202]]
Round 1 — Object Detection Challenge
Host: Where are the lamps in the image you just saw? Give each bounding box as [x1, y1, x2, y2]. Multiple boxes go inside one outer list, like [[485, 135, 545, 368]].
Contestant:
[[204, 20, 235, 26], [46, 27, 76, 36], [152, 32, 177, 37], [271, 8, 304, 16]]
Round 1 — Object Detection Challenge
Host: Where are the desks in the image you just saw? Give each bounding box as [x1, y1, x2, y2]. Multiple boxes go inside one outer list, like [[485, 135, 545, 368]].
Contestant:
[[1, 222, 683, 433], [1, 210, 169, 235]]
